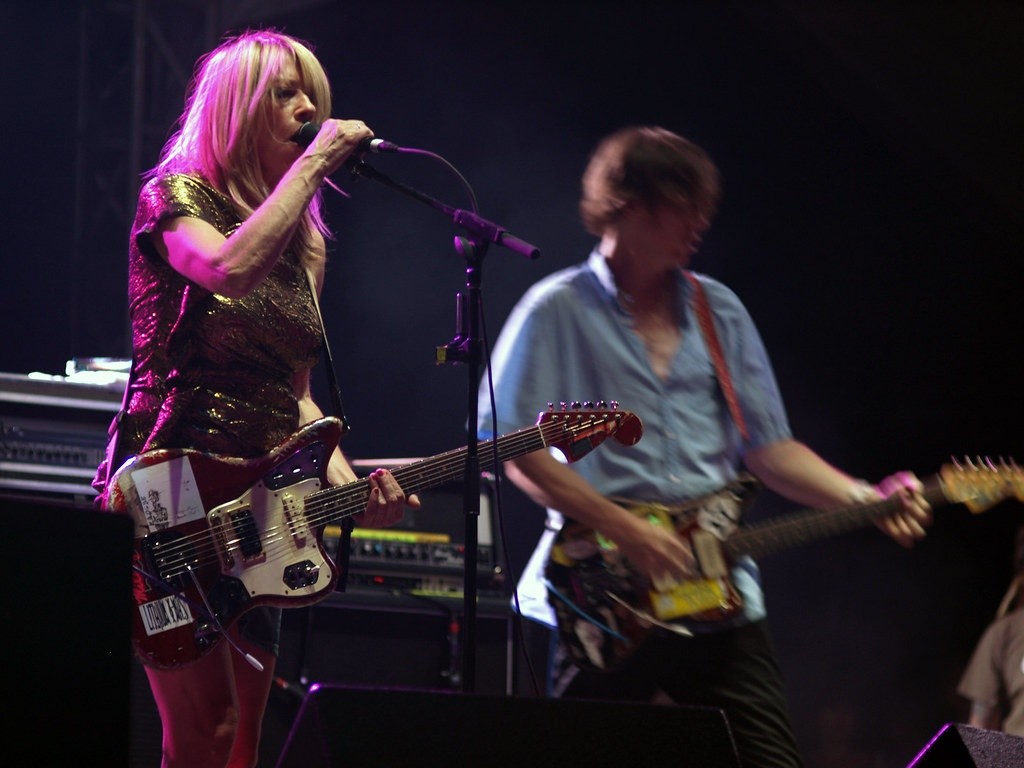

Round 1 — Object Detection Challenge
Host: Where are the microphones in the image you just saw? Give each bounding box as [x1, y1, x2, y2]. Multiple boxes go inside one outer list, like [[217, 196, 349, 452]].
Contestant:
[[297, 122, 398, 154]]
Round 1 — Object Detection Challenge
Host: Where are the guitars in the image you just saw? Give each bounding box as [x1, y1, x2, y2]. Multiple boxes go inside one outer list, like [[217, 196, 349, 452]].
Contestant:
[[543, 454, 1024, 673], [107, 400, 644, 671]]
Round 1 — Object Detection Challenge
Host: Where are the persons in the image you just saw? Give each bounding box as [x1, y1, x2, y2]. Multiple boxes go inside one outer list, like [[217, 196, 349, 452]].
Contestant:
[[957, 525, 1024, 736], [464, 123, 934, 768], [88, 25, 422, 768]]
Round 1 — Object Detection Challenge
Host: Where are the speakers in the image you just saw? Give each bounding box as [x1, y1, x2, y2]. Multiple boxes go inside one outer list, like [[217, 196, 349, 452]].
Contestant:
[[293, 605, 519, 696], [275, 685, 743, 768], [0, 493, 137, 768], [908, 722, 1024, 768]]
